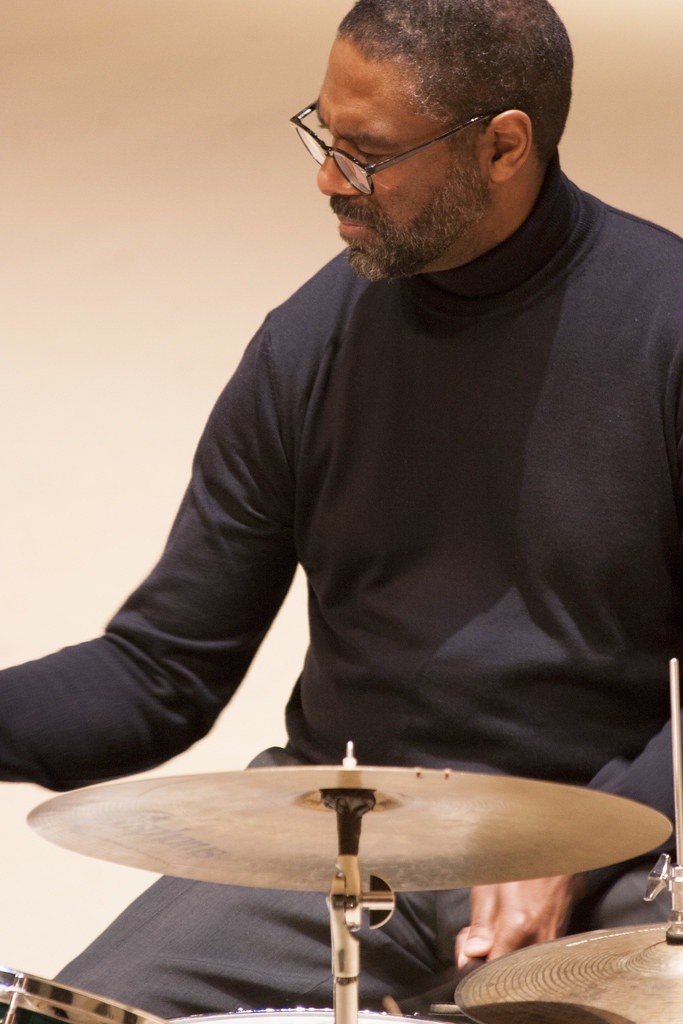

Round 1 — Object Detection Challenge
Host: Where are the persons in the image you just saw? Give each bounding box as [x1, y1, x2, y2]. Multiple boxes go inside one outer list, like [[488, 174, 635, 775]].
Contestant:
[[0, 0, 683, 1018]]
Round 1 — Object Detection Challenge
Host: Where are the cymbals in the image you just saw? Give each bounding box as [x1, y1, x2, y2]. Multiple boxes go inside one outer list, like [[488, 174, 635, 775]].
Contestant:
[[455, 923, 682, 1023], [26, 741, 673, 893]]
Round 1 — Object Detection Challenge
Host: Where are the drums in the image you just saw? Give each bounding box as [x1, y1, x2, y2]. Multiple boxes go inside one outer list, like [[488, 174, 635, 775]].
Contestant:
[[0, 965, 165, 1024]]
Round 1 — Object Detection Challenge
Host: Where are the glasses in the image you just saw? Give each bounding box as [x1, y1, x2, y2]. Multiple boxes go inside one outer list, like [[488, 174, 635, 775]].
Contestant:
[[289, 100, 498, 195]]
